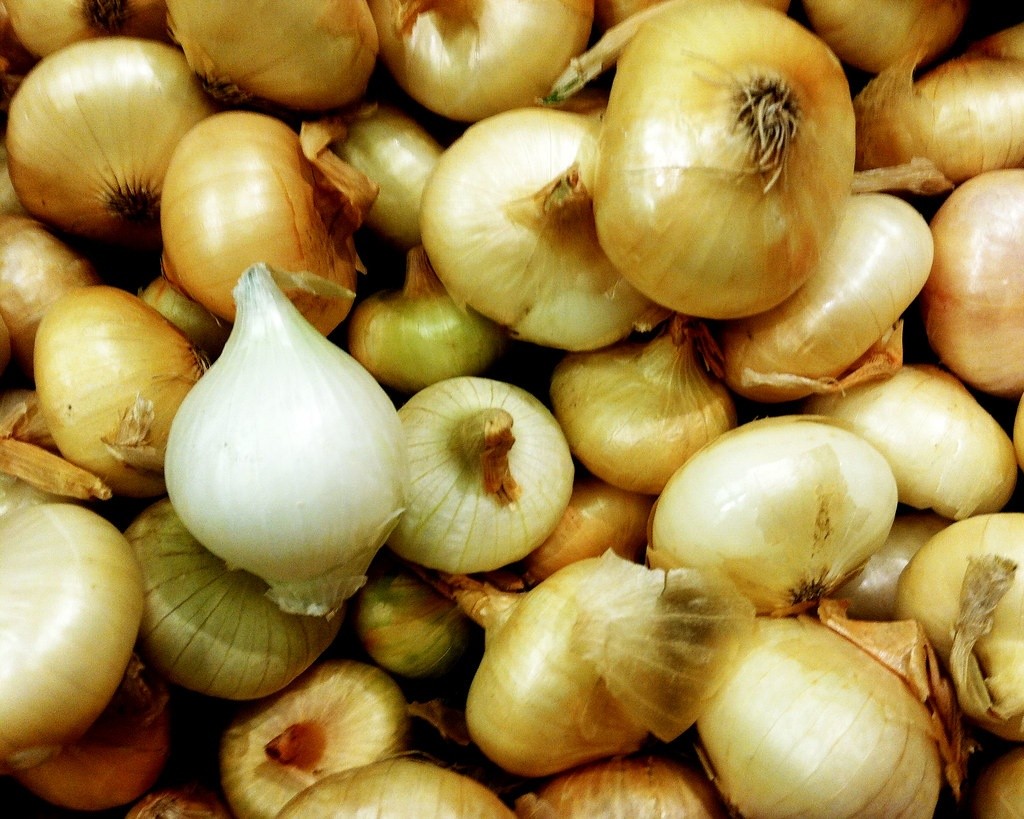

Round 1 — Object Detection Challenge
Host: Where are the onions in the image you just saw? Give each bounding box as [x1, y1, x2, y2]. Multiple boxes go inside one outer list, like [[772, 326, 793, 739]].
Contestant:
[[0, 1, 1024, 819]]
